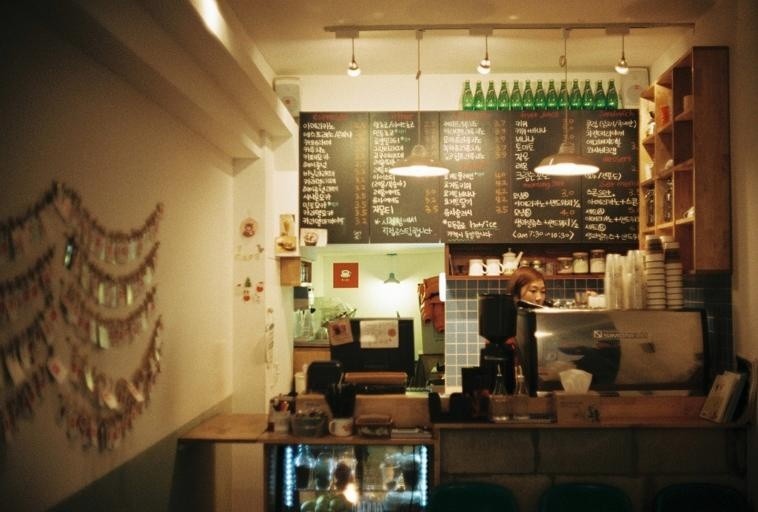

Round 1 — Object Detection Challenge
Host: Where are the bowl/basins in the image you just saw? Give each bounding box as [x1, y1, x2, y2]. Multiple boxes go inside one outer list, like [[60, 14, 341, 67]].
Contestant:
[[557, 369, 593, 396]]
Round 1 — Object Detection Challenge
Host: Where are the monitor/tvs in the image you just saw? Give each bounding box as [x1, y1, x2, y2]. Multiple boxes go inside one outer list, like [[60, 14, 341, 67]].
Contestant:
[[328, 317, 415, 379]]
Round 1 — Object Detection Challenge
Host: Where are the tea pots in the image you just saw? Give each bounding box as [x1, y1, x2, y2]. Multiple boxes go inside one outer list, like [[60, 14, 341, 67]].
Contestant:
[[500, 247, 524, 277]]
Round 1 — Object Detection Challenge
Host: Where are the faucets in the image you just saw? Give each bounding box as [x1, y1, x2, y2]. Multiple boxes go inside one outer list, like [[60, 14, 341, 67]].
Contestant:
[[322, 311, 347, 328]]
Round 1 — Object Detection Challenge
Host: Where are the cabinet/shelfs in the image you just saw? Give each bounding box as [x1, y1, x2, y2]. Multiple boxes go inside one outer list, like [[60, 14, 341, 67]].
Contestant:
[[262, 438, 435, 509], [638, 45, 732, 274]]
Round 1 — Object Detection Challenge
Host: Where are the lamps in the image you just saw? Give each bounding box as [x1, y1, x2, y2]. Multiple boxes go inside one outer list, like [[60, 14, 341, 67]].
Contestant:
[[476, 28, 494, 75], [615, 31, 632, 76], [532, 28, 601, 177], [347, 23, 361, 78], [386, 22, 451, 178]]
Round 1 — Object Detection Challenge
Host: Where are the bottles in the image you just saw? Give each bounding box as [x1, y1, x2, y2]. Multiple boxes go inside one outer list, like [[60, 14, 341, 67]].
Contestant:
[[663, 188, 672, 224], [513, 375, 533, 420], [490, 373, 511, 421], [647, 192, 654, 227], [266, 446, 426, 510], [458, 78, 617, 110]]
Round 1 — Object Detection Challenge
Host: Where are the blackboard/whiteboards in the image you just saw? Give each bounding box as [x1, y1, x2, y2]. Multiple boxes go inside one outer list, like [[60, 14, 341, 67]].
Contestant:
[[299, 108, 640, 245]]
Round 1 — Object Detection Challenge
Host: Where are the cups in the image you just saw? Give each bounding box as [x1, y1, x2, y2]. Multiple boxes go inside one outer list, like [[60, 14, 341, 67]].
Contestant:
[[295, 303, 329, 345], [468, 258, 490, 277], [603, 235, 690, 312], [486, 258, 506, 278], [302, 232, 318, 245], [272, 409, 291, 436], [327, 416, 356, 437], [680, 204, 694, 220]]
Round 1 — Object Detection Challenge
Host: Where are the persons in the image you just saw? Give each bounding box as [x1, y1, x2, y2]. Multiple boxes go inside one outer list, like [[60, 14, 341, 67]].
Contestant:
[[242, 223, 254, 237], [487, 265, 556, 368]]
